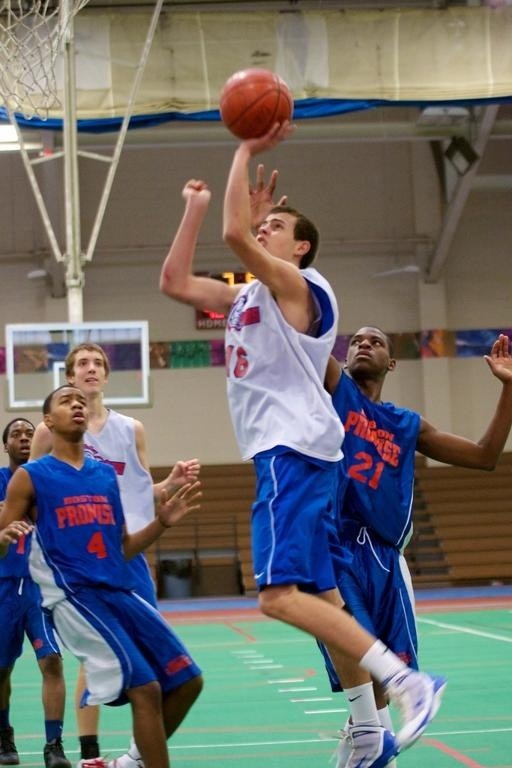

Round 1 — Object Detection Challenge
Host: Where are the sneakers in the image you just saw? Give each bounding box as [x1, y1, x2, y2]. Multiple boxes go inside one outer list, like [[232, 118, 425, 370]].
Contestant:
[[109, 751, 145, 768], [77, 756, 107, 768], [0, 725, 20, 766], [45, 740, 72, 767], [342, 723, 403, 768], [393, 666, 449, 754]]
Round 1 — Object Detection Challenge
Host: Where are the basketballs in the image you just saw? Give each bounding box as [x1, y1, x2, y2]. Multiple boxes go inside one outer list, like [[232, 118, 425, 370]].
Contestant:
[[217, 66, 296, 140]]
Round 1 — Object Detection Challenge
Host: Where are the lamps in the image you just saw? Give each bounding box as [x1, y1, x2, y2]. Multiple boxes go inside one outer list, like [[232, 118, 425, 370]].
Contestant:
[[445, 137, 478, 177]]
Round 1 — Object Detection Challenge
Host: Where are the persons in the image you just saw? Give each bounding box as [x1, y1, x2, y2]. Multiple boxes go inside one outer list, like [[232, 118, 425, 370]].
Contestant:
[[160, 122, 450, 768], [236, 162, 511, 768], [2, 418, 66, 768], [24, 344, 203, 768], [3, 387, 208, 768]]
